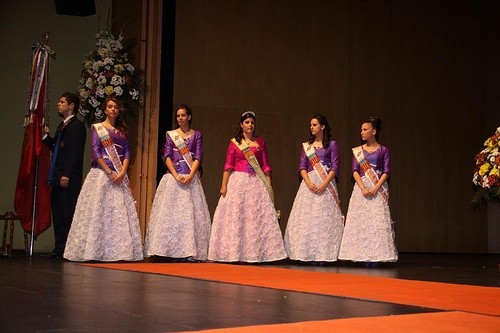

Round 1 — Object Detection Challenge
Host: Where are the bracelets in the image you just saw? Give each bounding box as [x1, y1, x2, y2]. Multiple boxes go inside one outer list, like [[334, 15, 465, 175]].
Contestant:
[[176, 175, 180, 180], [107, 171, 112, 176]]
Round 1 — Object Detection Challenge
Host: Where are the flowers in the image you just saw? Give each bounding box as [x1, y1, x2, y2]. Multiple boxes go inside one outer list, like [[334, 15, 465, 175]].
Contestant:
[[465, 126, 500, 211], [76, 29, 142, 125]]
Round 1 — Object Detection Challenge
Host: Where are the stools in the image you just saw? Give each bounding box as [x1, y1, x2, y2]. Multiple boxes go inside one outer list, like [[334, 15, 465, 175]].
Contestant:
[[0, 212, 30, 258]]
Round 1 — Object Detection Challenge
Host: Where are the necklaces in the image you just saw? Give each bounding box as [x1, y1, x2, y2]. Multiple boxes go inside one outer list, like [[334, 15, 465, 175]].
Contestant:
[[317, 146, 321, 149]]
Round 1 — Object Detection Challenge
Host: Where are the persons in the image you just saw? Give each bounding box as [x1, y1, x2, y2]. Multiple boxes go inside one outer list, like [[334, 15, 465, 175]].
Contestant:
[[282, 114, 344, 262], [41, 92, 86, 261], [337, 117, 398, 262], [144, 104, 212, 262], [62, 97, 144, 263], [208, 112, 287, 263]]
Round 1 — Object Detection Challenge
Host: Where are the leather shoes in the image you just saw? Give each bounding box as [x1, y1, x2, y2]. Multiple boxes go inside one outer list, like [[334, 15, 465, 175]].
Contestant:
[[40, 249, 62, 258]]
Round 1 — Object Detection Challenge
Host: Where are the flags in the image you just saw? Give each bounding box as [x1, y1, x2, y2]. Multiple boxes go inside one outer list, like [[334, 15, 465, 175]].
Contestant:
[[14, 45, 52, 241]]
